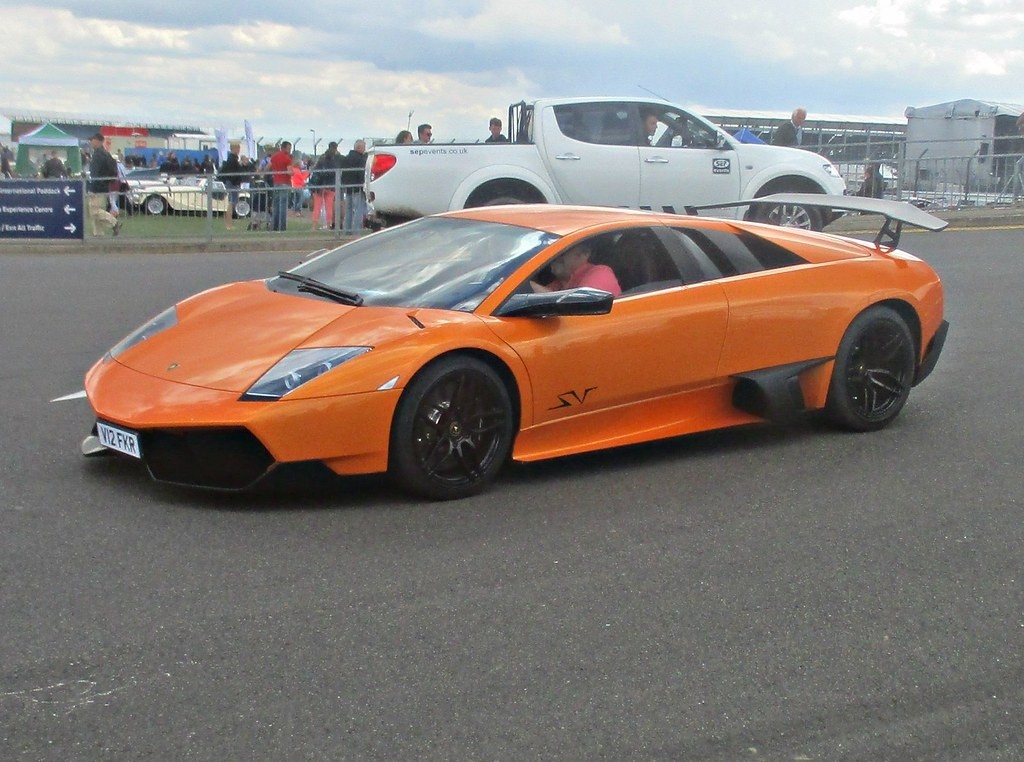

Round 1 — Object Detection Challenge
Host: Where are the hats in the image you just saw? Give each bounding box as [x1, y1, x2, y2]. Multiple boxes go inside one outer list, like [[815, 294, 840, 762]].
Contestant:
[[86, 132, 106, 142]]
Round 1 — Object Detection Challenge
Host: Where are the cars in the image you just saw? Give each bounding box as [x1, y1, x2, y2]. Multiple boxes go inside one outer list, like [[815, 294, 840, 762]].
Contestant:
[[84, 193, 949, 501], [121, 172, 251, 218]]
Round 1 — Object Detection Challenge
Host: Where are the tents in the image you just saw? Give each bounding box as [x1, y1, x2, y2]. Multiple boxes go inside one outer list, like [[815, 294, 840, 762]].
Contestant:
[[14, 121, 82, 175]]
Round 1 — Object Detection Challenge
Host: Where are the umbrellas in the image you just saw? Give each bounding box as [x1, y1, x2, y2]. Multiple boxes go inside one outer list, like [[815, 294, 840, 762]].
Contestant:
[[722, 126, 768, 149]]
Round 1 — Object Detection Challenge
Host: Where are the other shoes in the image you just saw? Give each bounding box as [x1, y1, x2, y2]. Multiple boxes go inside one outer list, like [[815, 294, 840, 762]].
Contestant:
[[111, 220, 124, 237]]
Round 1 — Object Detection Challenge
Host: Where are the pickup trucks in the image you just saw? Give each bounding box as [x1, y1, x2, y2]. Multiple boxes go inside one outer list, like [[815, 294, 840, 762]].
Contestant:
[[363, 97, 849, 233]]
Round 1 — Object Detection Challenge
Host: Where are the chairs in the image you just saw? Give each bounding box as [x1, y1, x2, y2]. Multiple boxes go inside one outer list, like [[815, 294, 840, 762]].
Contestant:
[[613, 231, 673, 294]]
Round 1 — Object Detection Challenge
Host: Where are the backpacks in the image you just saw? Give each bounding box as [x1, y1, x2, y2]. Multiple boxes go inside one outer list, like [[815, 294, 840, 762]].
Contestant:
[[93, 150, 118, 182]]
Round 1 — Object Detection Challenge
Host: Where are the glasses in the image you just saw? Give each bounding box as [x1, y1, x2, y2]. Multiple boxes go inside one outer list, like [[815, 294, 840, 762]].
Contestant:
[[421, 132, 433, 136]]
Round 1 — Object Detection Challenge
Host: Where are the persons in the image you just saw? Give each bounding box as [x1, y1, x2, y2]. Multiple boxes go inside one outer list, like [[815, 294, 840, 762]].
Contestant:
[[309, 141, 346, 232], [854, 161, 886, 215], [485, 118, 507, 143], [239, 154, 274, 219], [263, 142, 294, 231], [342, 139, 368, 238], [222, 143, 242, 230], [396, 130, 413, 143], [530, 238, 623, 300], [117, 148, 219, 176], [773, 108, 807, 149], [291, 161, 311, 217], [414, 124, 432, 143], [640, 108, 693, 148], [0, 139, 93, 180], [88, 132, 122, 238]]
